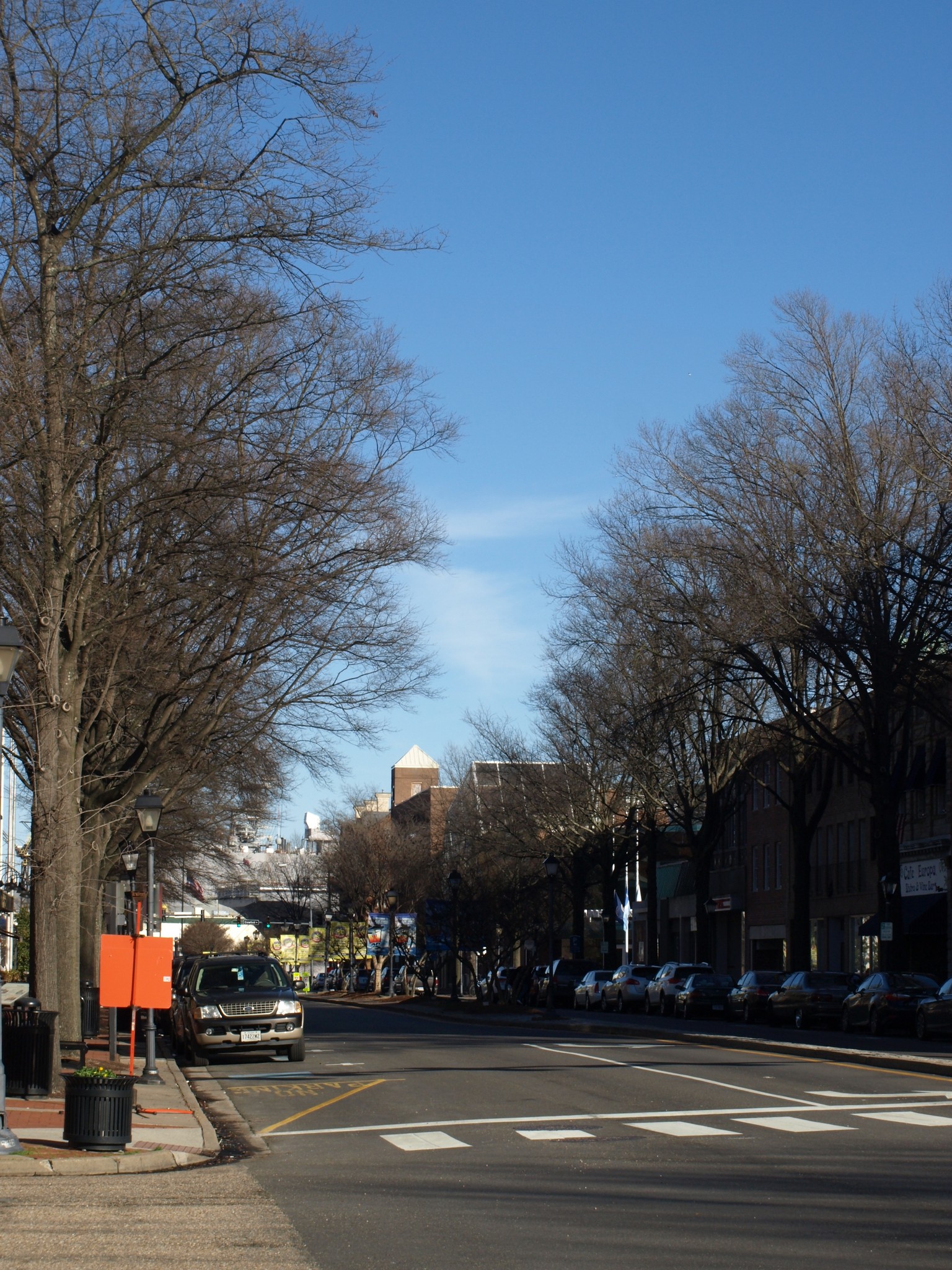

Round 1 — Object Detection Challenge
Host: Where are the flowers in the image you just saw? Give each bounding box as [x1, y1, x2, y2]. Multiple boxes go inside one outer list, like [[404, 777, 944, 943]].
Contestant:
[[73, 1065, 117, 1078]]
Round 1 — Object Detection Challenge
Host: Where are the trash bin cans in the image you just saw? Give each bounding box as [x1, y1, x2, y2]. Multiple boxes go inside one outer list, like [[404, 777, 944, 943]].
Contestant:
[[2, 997, 60, 1099], [80, 980, 101, 1039]]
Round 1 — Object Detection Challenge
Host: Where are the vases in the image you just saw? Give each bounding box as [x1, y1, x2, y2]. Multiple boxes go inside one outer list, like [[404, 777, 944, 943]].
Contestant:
[[58, 1071, 137, 1151]]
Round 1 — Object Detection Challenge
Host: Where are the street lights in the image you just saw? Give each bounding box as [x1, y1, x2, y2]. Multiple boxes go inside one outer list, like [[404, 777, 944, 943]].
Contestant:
[[322, 914, 333, 992], [446, 869, 462, 1000], [293, 919, 301, 972], [386, 888, 399, 997], [133, 788, 166, 1085], [118, 840, 141, 937], [880, 871, 899, 972], [598, 909, 612, 970], [703, 895, 719, 970]]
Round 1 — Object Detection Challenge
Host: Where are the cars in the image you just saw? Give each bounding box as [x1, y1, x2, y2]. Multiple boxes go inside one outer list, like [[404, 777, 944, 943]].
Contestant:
[[571, 969, 616, 1011], [675, 973, 737, 1020], [477, 965, 547, 1007], [727, 970, 952, 1043], [312, 967, 438, 999]]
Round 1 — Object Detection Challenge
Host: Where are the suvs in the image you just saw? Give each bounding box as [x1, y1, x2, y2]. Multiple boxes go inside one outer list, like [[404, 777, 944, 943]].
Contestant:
[[601, 960, 713, 1017], [165, 951, 306, 1065]]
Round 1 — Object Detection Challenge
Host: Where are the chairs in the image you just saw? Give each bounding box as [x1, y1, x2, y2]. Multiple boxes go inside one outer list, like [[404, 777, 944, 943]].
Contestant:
[[254, 970, 269, 984]]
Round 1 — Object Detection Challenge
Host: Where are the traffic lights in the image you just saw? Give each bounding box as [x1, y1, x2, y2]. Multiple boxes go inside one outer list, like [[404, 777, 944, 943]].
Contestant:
[[266, 917, 271, 927], [237, 916, 241, 927]]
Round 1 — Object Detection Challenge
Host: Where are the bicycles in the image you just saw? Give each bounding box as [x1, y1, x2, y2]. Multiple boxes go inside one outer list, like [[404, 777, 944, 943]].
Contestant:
[[468, 978, 480, 997]]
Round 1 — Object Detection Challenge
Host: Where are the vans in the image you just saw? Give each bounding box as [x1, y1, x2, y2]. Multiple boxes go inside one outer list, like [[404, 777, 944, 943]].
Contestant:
[[539, 957, 604, 1007]]
